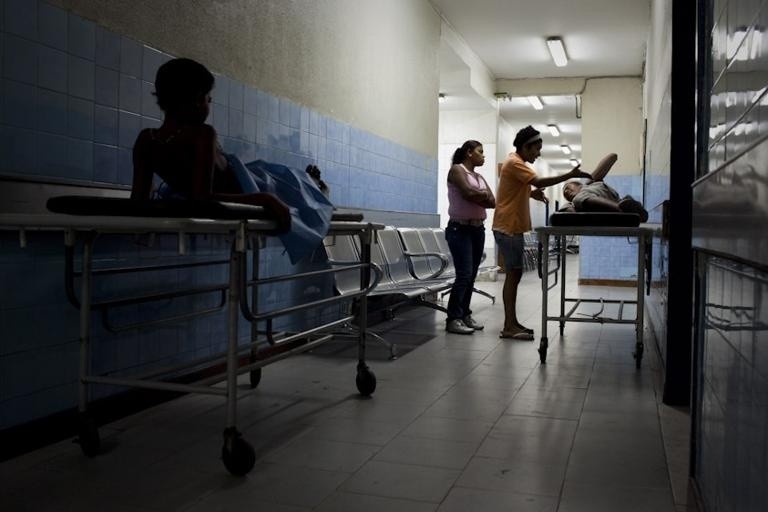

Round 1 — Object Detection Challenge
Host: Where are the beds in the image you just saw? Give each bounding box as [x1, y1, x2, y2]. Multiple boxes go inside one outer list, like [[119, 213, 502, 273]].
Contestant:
[[47, 196, 385, 479]]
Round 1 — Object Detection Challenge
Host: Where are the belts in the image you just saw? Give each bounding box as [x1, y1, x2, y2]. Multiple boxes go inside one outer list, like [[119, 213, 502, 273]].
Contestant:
[[460, 220, 483, 227]]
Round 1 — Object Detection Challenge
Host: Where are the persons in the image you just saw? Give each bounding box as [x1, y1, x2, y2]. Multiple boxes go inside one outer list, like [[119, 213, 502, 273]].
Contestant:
[[561, 153, 649, 223], [491, 125, 593, 341], [129, 56, 329, 235], [445, 139, 496, 335]]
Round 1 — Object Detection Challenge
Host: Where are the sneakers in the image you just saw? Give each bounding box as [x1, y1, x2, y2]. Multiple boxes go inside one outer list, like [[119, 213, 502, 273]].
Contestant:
[[464, 315, 483, 330], [446, 318, 474, 334]]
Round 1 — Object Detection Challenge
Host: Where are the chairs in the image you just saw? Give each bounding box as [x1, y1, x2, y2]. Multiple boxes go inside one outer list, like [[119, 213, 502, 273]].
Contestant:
[[523, 232, 579, 271], [307, 227, 495, 360]]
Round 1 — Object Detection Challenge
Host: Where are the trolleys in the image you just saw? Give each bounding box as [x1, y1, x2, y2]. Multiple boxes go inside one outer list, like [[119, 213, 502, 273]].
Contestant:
[[0, 209, 386, 478]]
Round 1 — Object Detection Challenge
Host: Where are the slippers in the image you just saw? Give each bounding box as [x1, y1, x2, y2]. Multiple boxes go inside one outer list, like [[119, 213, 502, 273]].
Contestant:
[[516, 322, 533, 334], [498, 328, 532, 340], [618, 199, 648, 223]]
